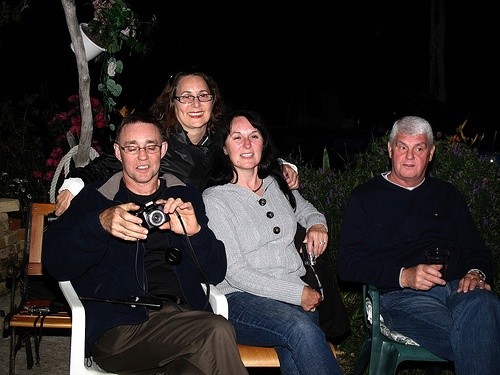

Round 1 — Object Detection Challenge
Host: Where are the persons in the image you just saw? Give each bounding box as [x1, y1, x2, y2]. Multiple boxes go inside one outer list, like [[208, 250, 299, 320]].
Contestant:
[[54, 67, 300, 217], [343, 116, 500, 372], [202, 111, 342, 374], [41, 114, 248, 375]]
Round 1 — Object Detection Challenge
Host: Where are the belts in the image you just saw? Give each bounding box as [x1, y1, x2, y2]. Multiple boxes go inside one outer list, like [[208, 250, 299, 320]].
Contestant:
[[149, 294, 187, 305]]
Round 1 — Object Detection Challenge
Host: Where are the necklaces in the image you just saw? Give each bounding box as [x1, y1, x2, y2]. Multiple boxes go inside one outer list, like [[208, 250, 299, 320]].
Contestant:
[[252, 179, 263, 193]]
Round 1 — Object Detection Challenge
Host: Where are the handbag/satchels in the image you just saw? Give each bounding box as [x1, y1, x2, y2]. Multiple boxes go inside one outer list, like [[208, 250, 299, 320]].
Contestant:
[[303, 263, 351, 346]]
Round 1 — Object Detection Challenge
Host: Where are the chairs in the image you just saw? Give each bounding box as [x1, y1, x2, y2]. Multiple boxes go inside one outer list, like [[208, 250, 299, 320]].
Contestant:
[[353, 283, 449, 375], [58, 281, 228, 375], [3, 201, 72, 375]]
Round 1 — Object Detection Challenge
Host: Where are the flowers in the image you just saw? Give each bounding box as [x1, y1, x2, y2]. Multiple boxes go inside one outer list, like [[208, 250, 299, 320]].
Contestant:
[[87, 0, 156, 131]]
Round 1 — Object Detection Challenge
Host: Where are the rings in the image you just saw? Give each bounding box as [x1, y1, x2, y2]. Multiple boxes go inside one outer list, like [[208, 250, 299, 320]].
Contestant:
[[319, 241, 325, 245]]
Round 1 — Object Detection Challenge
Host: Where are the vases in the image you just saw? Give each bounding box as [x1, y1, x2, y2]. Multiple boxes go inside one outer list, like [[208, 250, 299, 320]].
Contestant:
[[70, 23, 107, 62]]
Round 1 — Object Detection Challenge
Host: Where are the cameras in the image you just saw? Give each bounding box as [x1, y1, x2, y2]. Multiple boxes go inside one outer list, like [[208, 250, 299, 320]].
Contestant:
[[302, 243, 316, 266], [134, 204, 170, 232]]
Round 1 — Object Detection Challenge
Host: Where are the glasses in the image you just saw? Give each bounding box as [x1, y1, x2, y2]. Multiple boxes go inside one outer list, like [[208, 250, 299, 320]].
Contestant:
[[119, 144, 162, 155], [174, 93, 213, 104]]
